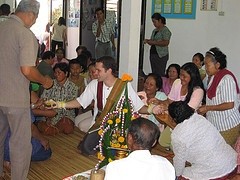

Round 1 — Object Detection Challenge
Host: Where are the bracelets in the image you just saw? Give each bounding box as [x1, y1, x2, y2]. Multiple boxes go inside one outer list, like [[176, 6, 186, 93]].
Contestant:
[[205, 106, 208, 111]]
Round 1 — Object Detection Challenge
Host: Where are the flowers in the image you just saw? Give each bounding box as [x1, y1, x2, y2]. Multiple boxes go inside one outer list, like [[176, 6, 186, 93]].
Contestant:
[[97, 74, 136, 171]]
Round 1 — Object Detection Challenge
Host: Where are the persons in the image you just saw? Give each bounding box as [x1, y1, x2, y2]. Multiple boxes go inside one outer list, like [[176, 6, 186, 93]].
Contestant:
[[92, 8, 116, 58], [0, 0, 54, 180], [143, 13, 171, 77], [0, 4, 11, 22], [51, 17, 68, 54], [4, 23, 240, 180]]
